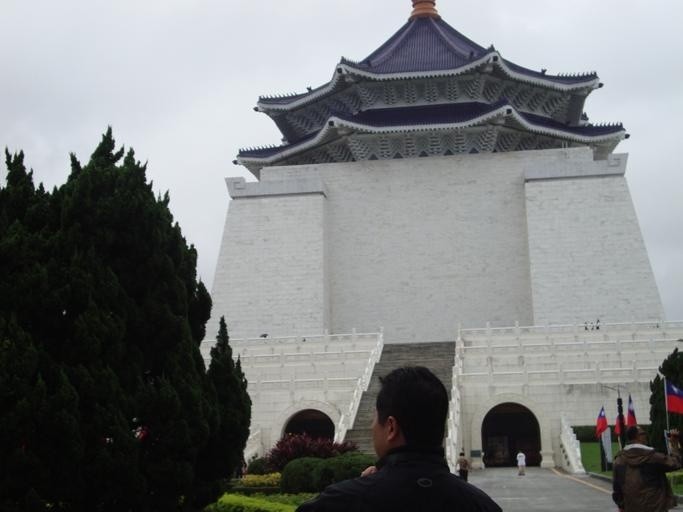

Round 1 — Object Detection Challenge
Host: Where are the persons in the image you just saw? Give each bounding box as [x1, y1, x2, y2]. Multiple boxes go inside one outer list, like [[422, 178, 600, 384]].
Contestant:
[[610, 425, 683, 512], [454, 452, 470, 481], [291, 361, 503, 512], [516, 449, 526, 476]]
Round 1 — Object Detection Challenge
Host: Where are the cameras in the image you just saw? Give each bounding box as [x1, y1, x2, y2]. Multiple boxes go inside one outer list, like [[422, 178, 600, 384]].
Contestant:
[[664, 429, 670, 439]]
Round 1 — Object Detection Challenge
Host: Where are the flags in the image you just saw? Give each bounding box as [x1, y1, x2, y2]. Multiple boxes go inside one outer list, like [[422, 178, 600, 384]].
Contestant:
[[626, 393, 637, 429], [594, 405, 607, 439], [664, 376, 683, 414], [614, 393, 625, 435]]
